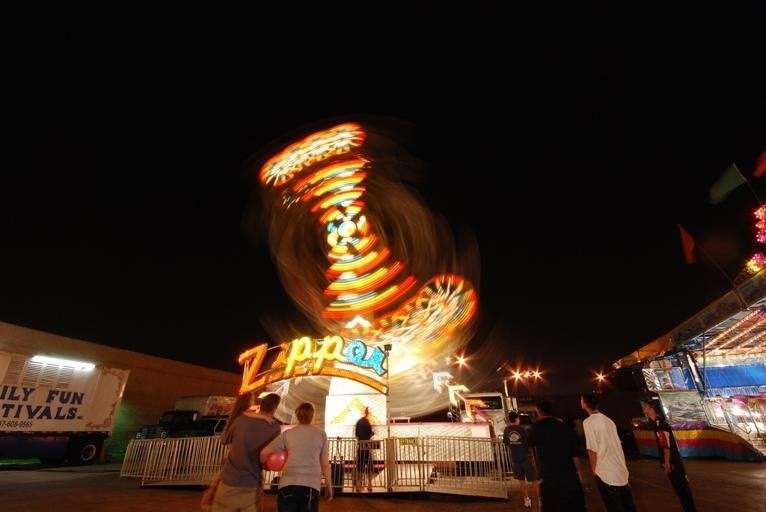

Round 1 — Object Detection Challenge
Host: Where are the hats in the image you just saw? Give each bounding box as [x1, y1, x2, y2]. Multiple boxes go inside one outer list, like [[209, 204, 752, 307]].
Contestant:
[[641, 398, 659, 408]]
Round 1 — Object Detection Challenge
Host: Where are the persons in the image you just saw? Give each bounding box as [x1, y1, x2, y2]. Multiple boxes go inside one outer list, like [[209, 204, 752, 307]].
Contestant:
[[643, 400, 696, 512], [210, 392, 281, 511], [579, 394, 637, 512], [528, 399, 588, 512], [503, 412, 542, 507], [201, 393, 275, 506], [260, 402, 333, 512], [355, 406, 375, 491]]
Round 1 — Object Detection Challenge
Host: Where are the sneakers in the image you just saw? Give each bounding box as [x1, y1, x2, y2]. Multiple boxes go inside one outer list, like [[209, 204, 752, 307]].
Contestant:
[[201, 489, 212, 510], [524, 500, 531, 507]]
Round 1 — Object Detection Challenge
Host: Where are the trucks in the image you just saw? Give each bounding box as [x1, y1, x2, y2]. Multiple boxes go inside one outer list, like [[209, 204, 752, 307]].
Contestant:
[[0, 351, 130, 465], [447, 392, 507, 439], [136, 410, 229, 439]]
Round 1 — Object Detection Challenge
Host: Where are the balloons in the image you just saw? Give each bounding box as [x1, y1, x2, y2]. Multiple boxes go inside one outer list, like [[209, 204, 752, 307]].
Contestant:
[[266, 447, 287, 473]]
